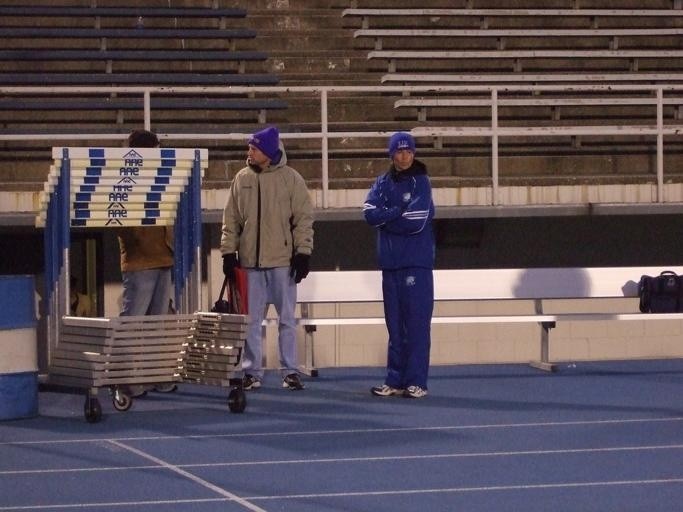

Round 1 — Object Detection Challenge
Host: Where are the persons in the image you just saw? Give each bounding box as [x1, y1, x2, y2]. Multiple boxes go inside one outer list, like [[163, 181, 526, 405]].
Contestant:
[[221, 127, 315, 389], [116, 130, 177, 398], [364, 132, 435, 397]]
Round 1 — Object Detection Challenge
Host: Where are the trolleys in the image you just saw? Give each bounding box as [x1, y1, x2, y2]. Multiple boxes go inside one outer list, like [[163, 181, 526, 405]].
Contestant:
[[35, 147, 252, 423]]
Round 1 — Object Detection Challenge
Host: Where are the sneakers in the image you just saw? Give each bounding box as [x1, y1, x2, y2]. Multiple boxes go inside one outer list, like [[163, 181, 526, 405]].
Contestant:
[[406, 386, 428, 397], [243, 374, 261, 390], [371, 384, 404, 396], [145, 382, 177, 393], [282, 375, 305, 391], [128, 385, 146, 398]]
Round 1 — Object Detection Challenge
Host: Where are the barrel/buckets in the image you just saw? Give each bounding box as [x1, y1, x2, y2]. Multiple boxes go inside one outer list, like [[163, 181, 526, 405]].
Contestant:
[[1, 275, 40, 422]]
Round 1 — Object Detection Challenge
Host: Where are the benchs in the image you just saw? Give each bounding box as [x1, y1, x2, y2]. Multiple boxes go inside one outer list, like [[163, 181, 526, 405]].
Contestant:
[[1, 2, 304, 150], [340, 0, 683, 149]]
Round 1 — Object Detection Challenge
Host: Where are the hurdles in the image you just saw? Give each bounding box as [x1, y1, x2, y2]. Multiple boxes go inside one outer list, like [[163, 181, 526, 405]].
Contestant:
[[35, 147, 251, 388]]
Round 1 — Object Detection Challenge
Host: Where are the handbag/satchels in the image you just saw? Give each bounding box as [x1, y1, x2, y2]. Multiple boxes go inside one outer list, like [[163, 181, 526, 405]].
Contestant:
[[639, 271, 683, 313], [210, 301, 245, 371]]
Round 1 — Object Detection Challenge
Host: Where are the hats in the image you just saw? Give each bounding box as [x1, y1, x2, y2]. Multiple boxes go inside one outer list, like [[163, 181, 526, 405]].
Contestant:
[[248, 128, 279, 158], [128, 130, 158, 147], [389, 134, 416, 156]]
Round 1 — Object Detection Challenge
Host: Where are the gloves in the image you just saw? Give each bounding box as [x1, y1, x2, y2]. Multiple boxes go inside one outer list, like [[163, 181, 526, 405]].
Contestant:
[[290, 253, 310, 282], [222, 254, 238, 282]]
